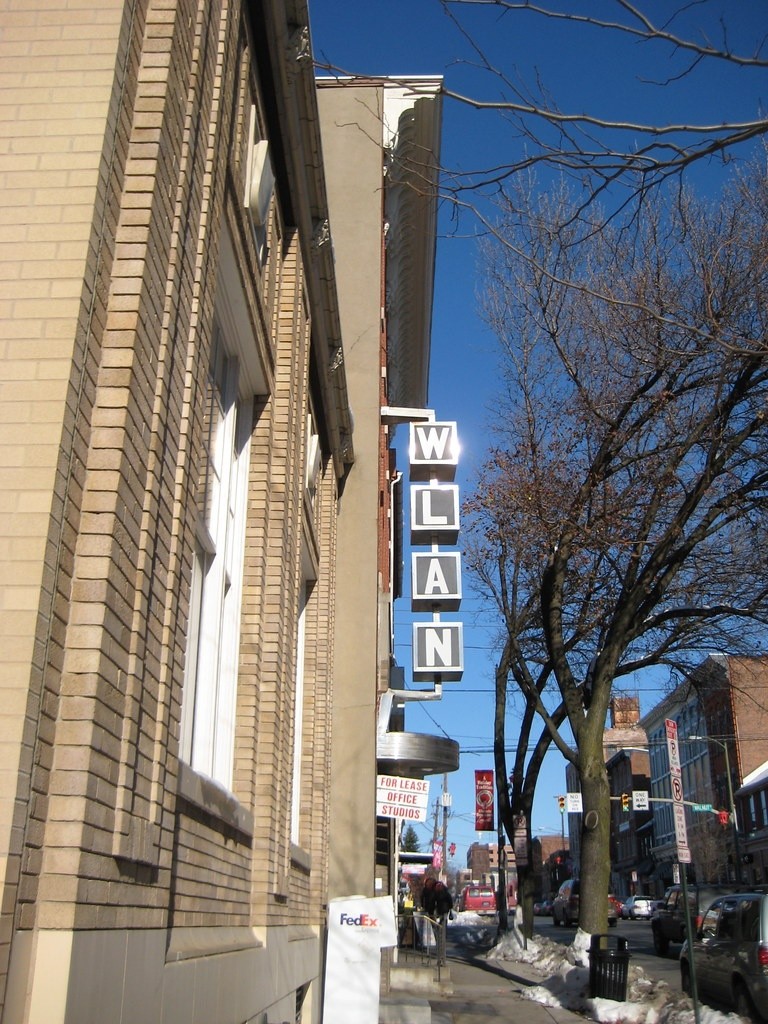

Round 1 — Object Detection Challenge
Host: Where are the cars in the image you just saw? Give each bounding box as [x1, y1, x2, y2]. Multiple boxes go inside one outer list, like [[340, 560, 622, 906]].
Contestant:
[[619, 895, 654, 920], [533, 900, 554, 916]]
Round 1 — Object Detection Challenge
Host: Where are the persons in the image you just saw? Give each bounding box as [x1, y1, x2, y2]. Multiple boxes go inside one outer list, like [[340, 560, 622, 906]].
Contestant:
[[420, 877, 453, 931]]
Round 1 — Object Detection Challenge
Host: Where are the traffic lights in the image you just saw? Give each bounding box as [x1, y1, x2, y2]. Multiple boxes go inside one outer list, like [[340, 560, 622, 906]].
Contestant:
[[621, 792, 630, 813], [559, 795, 566, 814]]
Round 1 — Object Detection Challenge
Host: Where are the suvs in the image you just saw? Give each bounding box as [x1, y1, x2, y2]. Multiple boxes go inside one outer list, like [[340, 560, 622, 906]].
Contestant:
[[649, 883, 730, 956], [550, 878, 618, 928]]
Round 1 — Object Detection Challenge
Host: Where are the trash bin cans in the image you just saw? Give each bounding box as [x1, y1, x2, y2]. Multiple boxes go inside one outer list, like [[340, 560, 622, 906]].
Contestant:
[[585, 933, 632, 1002]]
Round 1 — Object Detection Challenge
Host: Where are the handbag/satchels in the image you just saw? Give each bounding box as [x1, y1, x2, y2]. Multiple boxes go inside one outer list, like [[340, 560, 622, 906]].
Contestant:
[[449, 909, 453, 920]]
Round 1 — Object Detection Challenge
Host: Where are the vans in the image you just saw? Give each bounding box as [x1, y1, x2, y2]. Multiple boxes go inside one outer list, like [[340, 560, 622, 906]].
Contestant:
[[677, 893, 768, 1024], [457, 886, 497, 919]]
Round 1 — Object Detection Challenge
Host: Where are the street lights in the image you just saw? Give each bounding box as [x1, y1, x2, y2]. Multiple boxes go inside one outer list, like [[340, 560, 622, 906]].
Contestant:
[[689, 734, 745, 886]]
